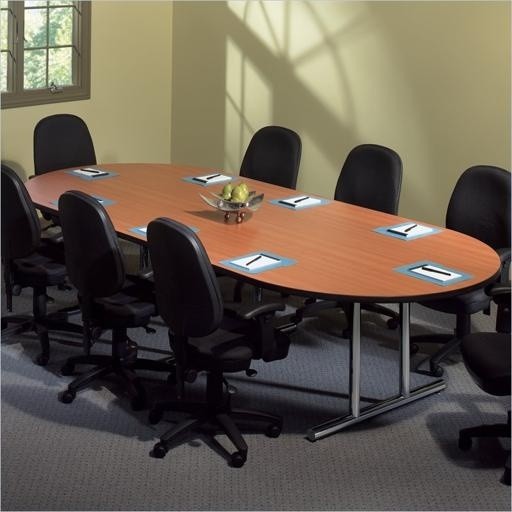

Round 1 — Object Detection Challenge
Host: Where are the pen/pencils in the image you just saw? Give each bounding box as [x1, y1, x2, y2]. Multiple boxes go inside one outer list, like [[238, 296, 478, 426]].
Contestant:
[[405, 224, 417, 232], [422, 266, 451, 276], [295, 197, 309, 203], [207, 174, 220, 179], [246, 256, 261, 265], [80, 169, 99, 173]]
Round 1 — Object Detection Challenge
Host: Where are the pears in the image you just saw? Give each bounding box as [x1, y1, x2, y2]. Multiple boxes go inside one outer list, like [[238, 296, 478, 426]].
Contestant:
[[223, 183, 249, 203]]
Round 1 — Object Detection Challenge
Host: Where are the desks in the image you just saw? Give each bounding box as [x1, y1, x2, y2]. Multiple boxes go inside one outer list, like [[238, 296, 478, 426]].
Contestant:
[[20, 162, 503, 444]]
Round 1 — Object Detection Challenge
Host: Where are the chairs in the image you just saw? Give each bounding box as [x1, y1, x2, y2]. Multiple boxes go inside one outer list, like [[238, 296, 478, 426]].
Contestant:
[[27, 111, 100, 295], [456, 277, 511, 487], [54, 186, 203, 415], [406, 165, 511, 381], [296, 142, 404, 346], [141, 215, 294, 469], [215, 126, 303, 307], [2, 162, 103, 369]]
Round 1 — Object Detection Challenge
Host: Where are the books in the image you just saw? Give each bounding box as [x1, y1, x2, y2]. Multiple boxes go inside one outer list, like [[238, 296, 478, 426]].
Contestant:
[[387, 223, 430, 237], [278, 195, 318, 207], [194, 172, 231, 183], [139, 226, 149, 233], [75, 166, 107, 178], [407, 262, 463, 282], [229, 253, 278, 269]]
